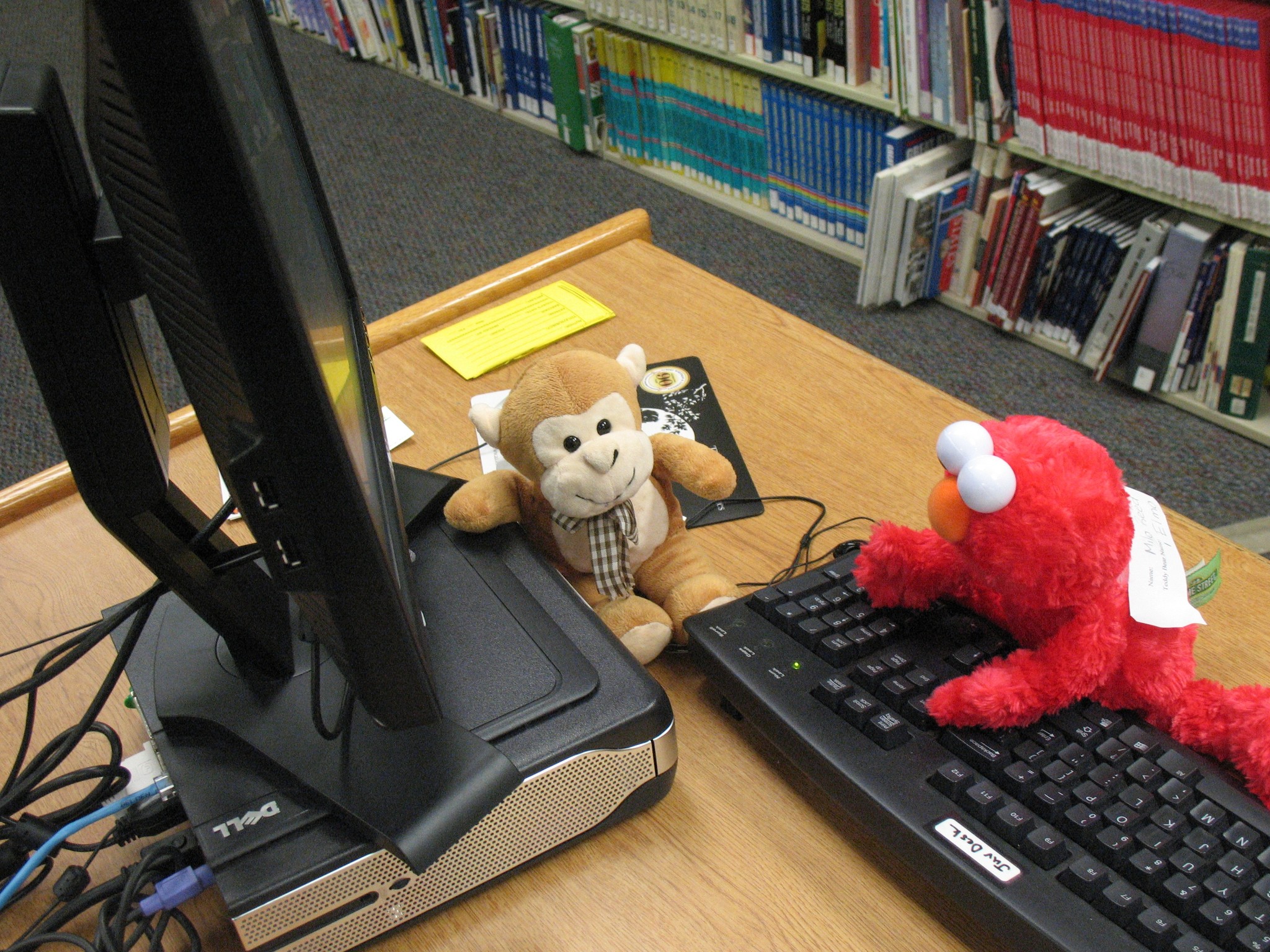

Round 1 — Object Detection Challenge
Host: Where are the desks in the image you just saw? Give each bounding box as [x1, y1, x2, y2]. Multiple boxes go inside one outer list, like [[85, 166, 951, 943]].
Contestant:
[[0, 209, 1270, 952]]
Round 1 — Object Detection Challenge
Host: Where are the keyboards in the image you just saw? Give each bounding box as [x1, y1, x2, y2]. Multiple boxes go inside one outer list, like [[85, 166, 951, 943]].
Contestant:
[[679, 549, 1270, 952]]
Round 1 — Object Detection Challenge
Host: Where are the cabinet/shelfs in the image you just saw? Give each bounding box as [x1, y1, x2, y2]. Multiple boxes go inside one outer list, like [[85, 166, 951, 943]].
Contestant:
[[260, 1, 1269, 449]]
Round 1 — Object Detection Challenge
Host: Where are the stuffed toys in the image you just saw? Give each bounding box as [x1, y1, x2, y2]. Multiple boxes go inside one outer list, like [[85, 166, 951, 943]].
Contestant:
[[442, 343, 744, 665], [853, 414, 1270, 811]]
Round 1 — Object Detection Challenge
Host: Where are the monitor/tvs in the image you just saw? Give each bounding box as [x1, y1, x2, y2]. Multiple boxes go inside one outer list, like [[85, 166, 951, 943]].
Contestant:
[[0, 0, 522, 879]]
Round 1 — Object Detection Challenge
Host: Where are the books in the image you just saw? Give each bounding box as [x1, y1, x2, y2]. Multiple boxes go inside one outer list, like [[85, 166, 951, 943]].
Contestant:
[[605, 0, 949, 307], [951, 0, 1270, 431], [261, 0, 604, 157]]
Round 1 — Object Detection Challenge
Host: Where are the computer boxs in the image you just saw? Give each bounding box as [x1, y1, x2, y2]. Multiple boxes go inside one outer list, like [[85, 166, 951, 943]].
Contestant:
[[96, 512, 681, 952]]
